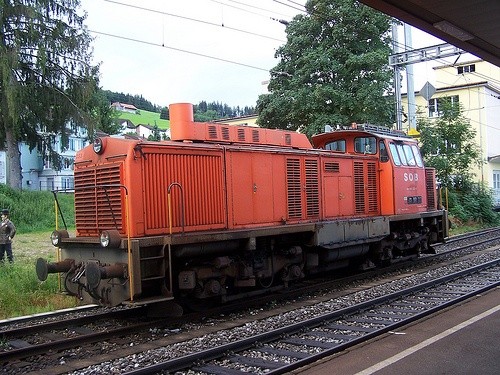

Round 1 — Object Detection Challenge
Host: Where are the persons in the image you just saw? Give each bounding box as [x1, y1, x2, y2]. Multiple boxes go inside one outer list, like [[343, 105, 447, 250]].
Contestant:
[[0, 209, 16, 269]]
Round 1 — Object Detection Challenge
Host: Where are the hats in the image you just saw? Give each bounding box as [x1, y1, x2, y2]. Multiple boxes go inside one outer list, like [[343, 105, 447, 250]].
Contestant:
[[0, 209, 9, 215]]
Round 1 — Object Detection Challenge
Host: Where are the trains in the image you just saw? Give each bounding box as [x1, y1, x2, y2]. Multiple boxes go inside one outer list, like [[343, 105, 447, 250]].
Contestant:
[[36, 102, 452, 310]]
